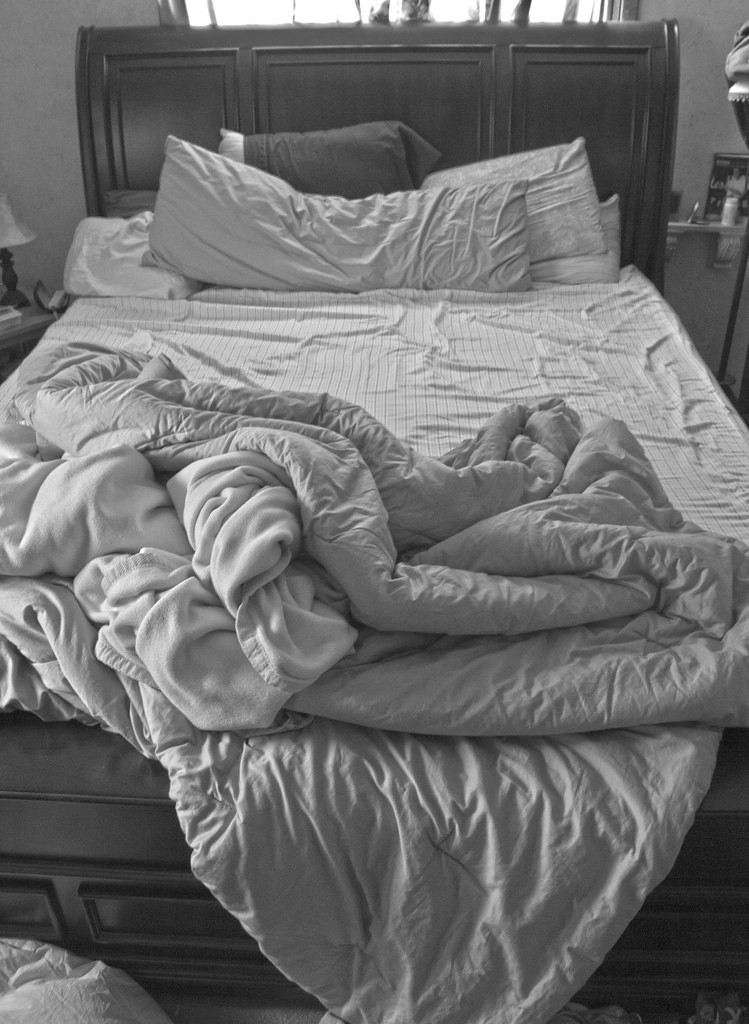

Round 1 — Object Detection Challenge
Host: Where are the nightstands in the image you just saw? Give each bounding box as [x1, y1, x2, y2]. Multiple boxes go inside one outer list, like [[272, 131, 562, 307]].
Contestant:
[[0, 310, 61, 386]]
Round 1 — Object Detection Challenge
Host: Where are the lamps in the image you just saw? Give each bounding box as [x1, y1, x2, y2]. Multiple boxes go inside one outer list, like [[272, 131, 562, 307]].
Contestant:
[[0, 194, 38, 308]]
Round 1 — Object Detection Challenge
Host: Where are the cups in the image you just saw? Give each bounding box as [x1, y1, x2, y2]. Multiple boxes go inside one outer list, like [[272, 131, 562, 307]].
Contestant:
[[722, 198, 738, 226]]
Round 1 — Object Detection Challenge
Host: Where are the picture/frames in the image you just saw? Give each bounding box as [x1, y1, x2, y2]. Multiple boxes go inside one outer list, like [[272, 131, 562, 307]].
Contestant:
[[704, 153, 749, 221]]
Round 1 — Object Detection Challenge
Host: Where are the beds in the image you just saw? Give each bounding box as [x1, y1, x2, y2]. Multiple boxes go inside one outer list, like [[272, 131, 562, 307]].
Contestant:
[[0, 18, 749, 1024]]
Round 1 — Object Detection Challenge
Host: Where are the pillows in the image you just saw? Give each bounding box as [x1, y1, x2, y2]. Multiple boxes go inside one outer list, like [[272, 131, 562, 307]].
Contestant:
[[218, 120, 441, 200], [530, 192, 622, 283], [63, 210, 212, 300], [141, 135, 528, 294], [418, 137, 608, 263]]
